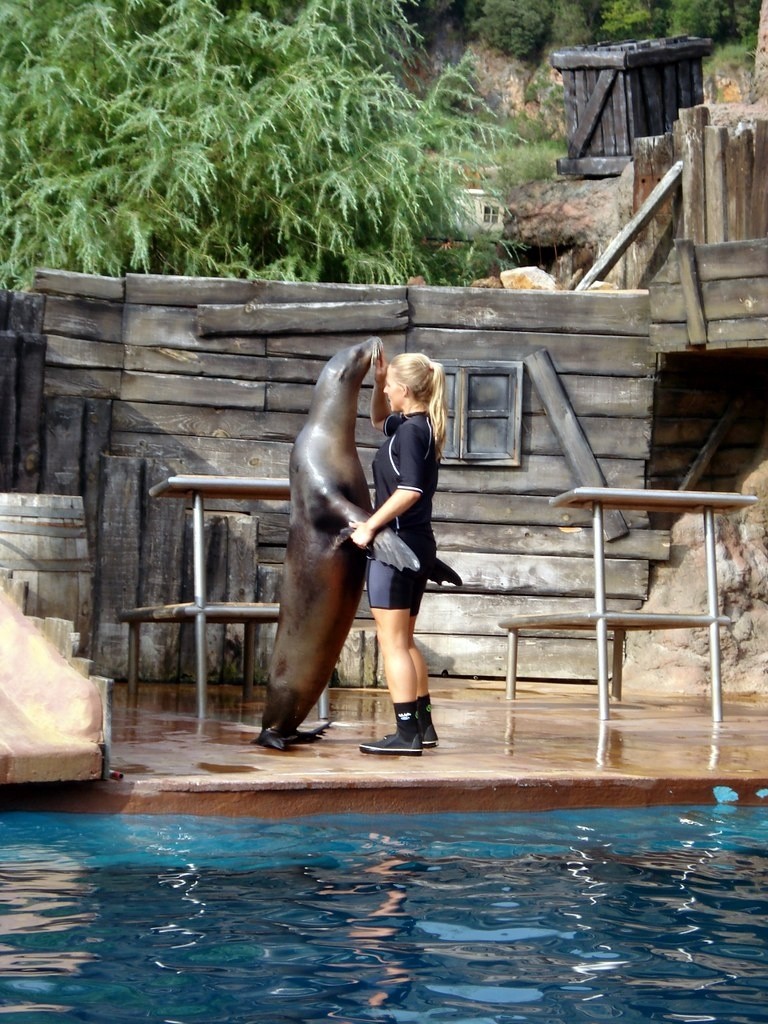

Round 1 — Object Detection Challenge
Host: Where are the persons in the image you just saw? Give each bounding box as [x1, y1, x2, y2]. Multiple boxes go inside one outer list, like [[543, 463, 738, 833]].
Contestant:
[[349, 346, 449, 757]]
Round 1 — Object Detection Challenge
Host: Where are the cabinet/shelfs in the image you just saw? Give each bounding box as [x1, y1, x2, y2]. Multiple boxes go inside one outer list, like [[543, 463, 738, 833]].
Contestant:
[[498, 486, 758, 724], [120, 473, 333, 718]]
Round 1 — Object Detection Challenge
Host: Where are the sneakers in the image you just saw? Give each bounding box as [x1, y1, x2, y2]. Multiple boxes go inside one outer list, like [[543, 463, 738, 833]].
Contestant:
[[359, 730, 422, 756], [383, 725, 438, 747]]
